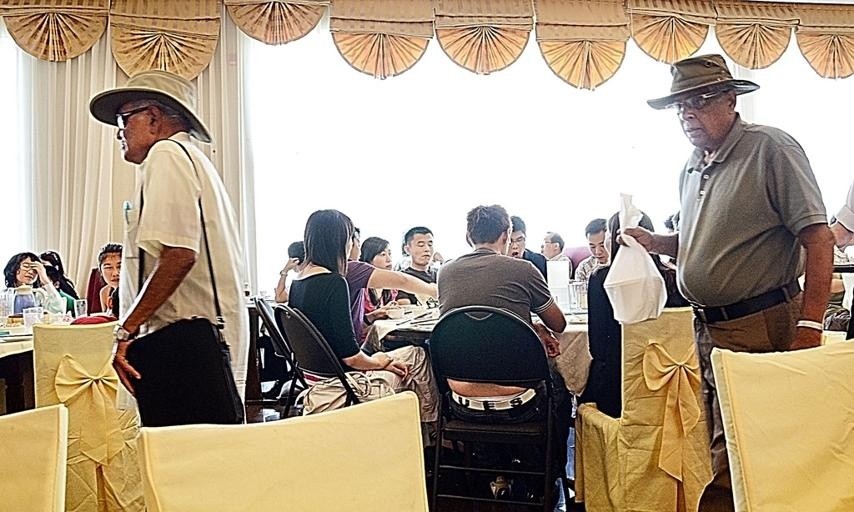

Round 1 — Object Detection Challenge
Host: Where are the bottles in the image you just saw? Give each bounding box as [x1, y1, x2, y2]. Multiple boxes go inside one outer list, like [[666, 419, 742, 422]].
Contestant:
[[243, 283, 251, 299]]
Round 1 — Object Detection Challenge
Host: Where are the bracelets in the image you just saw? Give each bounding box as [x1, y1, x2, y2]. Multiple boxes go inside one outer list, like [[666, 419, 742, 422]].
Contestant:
[[796, 320, 826, 331]]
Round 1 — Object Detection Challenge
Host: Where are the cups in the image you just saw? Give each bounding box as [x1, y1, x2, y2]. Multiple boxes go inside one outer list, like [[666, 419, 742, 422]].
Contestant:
[[569, 280, 588, 310], [13, 285, 46, 316], [74, 299, 88, 319], [22, 306, 45, 334]]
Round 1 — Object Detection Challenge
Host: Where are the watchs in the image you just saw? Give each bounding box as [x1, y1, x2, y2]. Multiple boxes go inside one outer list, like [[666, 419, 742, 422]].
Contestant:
[[115, 325, 135, 341]]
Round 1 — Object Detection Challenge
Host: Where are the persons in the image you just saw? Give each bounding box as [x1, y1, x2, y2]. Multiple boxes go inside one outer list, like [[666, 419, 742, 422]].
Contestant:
[[647, 53, 835, 512], [433, 207, 567, 498], [0, 243, 126, 318], [276, 182, 854, 336], [89, 68, 250, 424], [582, 211, 680, 421], [286, 209, 437, 444]]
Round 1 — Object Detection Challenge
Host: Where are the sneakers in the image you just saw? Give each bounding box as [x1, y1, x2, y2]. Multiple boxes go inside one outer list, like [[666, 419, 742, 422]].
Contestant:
[[490, 476, 509, 500]]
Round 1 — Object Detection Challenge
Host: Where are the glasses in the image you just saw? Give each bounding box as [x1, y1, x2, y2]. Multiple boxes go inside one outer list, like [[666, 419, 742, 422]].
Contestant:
[[115, 107, 149, 129], [667, 90, 721, 111]]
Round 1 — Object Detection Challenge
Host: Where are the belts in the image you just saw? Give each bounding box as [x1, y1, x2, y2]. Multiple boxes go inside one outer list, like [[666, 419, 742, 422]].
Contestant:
[[691, 280, 801, 322], [452, 388, 536, 410]]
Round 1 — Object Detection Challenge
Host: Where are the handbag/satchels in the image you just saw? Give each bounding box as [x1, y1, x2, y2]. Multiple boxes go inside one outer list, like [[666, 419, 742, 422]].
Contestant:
[[126, 317, 243, 427]]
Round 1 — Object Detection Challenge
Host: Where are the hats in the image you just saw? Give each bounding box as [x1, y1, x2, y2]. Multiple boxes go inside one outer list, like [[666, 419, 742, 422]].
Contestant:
[[90, 69, 213, 143], [647, 54, 760, 110]]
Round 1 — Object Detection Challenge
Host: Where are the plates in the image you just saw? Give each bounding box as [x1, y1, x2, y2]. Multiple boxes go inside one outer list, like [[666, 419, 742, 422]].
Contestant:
[[568, 315, 587, 324], [2, 336, 33, 342]]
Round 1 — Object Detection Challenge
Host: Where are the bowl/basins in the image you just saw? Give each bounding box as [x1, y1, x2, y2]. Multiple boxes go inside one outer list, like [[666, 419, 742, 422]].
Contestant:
[[387, 309, 404, 319]]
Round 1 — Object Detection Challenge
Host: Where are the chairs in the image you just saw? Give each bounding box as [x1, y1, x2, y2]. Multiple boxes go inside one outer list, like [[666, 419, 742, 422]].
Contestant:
[[254, 297, 312, 419], [709, 338, 854, 512], [273, 304, 364, 418], [1, 404, 71, 511], [576, 304, 716, 512], [424, 303, 569, 512], [4, 322, 126, 512], [137, 388, 434, 512]]
